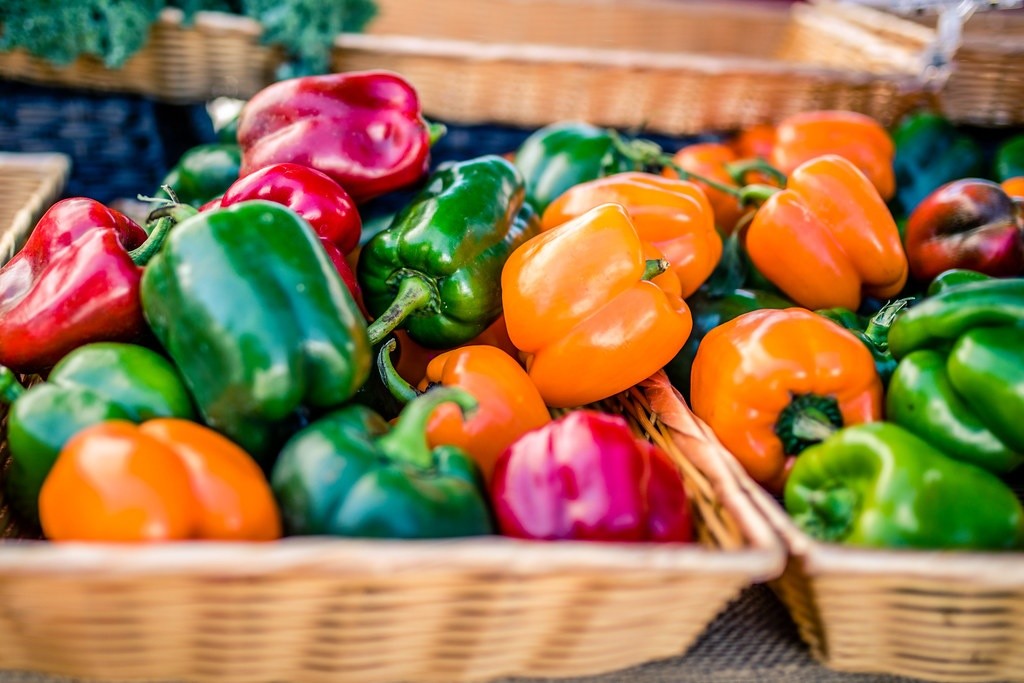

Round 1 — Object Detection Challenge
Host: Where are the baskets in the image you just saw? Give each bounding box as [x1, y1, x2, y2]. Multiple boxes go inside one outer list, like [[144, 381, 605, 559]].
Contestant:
[[0, 360, 782, 683], [0, 0, 1023, 141], [0, 152, 72, 265], [670, 384, 1024, 683]]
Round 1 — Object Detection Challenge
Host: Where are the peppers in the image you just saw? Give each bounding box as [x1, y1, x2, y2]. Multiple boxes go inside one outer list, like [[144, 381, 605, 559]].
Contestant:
[[0, 69, 1024, 550]]
